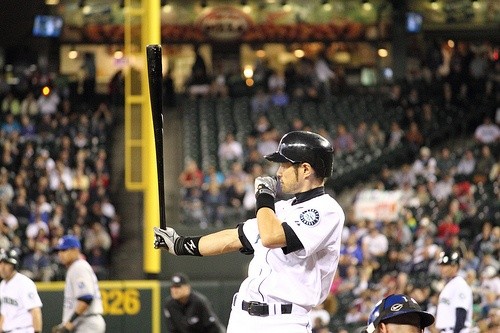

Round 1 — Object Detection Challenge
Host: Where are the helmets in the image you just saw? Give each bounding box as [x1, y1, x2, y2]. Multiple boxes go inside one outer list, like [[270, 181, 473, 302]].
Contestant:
[[368, 293, 434, 328], [0, 248, 18, 264], [435, 248, 462, 266], [263, 130, 335, 177]]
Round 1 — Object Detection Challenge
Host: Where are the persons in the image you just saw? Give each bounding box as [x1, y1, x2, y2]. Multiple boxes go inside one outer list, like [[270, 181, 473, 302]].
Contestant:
[[182, 42, 500, 333], [365, 294, 435, 333], [49, 234, 107, 333], [435, 251, 473, 333], [162, 273, 226, 333], [0, 114, 121, 284], [0, 249, 43, 333], [154, 130, 345, 333]]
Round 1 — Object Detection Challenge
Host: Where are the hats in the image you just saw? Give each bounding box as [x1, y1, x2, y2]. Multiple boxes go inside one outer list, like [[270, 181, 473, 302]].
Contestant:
[[48, 235, 81, 256], [169, 273, 189, 287]]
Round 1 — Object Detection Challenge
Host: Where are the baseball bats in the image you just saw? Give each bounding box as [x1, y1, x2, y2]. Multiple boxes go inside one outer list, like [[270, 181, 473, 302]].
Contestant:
[[147, 43, 169, 246]]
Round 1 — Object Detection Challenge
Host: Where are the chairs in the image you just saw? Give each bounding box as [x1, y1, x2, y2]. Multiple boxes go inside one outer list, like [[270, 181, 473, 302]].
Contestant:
[[183, 93, 390, 172]]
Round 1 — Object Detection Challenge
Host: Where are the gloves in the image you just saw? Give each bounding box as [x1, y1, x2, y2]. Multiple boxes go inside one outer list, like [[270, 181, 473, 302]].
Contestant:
[[254, 175, 277, 199], [152, 226, 180, 256]]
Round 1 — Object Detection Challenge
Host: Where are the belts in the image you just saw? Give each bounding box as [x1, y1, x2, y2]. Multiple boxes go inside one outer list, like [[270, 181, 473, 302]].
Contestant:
[[233, 296, 292, 317]]
